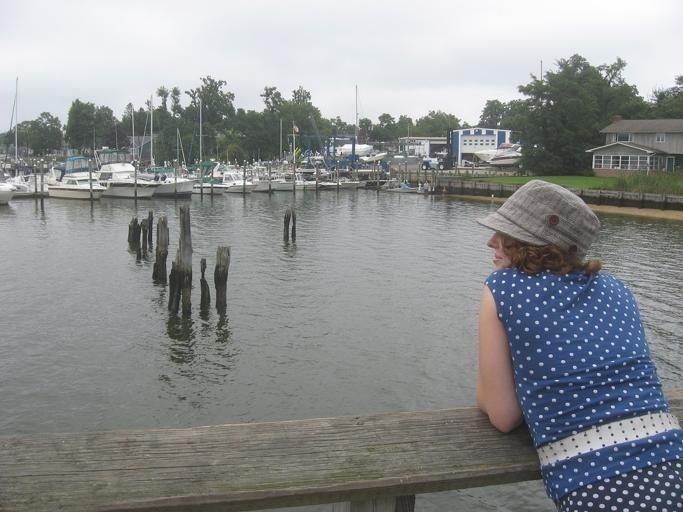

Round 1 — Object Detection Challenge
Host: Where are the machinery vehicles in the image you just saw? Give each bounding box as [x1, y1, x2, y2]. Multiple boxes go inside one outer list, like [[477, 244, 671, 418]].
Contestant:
[[421, 128, 455, 170]]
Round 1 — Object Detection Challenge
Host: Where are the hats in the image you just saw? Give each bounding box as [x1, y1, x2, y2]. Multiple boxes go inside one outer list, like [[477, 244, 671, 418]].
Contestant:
[[476, 180, 599, 260]]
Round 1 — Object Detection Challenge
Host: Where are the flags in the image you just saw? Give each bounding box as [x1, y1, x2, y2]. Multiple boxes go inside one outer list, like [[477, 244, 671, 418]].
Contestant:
[[294, 122, 299, 134]]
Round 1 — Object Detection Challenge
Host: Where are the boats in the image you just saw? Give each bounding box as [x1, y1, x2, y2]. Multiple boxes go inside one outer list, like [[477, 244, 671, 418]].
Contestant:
[[475, 141, 522, 165]]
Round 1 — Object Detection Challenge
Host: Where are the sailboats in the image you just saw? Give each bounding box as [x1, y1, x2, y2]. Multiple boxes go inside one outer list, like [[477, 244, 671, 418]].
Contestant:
[[1, 95, 384, 204]]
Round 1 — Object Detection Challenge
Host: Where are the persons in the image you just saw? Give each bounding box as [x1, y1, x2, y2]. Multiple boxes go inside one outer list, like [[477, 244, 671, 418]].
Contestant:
[[474, 179, 683, 512]]
